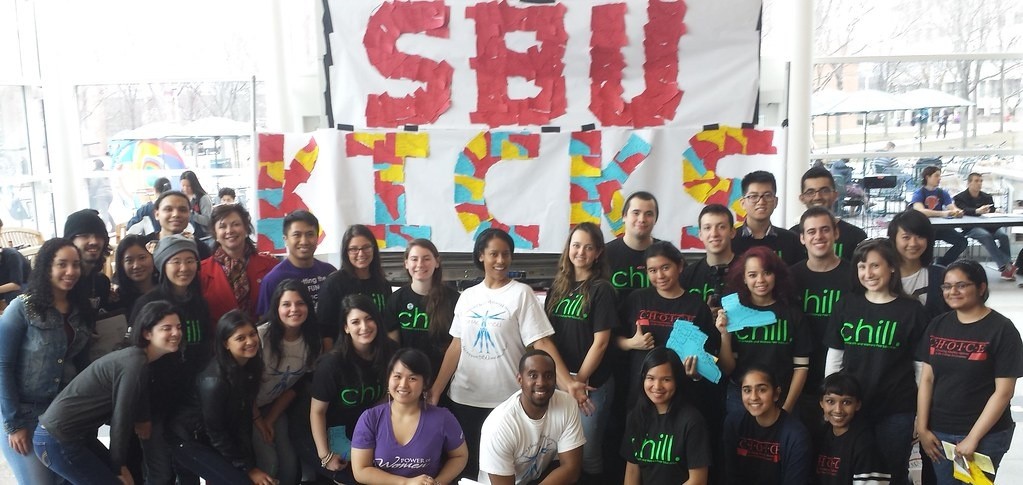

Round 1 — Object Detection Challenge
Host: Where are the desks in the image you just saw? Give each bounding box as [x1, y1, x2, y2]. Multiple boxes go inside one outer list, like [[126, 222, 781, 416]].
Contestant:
[[924, 213, 1023, 228]]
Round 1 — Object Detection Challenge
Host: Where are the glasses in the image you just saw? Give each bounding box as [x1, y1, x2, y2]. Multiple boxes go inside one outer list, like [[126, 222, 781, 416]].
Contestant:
[[743, 191, 776, 203], [802, 186, 835, 197], [856, 235, 890, 246], [940, 281, 975, 292], [345, 243, 375, 254], [167, 257, 198, 267]]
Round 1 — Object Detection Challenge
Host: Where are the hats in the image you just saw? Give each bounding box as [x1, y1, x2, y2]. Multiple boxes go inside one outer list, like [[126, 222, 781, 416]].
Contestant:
[[153, 233, 201, 278], [64, 209, 109, 244]]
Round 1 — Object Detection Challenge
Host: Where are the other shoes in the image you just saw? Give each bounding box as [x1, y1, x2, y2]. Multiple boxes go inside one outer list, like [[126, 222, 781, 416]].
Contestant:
[[298, 475, 323, 485], [1000, 264, 1019, 281], [332, 470, 362, 485]]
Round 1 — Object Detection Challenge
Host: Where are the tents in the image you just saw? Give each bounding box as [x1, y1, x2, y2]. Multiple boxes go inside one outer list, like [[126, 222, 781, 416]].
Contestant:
[[811, 87, 975, 199]]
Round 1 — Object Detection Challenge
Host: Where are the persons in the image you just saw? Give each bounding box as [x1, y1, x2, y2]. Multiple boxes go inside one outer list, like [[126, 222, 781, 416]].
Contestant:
[[605, 191, 691, 298], [952, 173, 1019, 280], [126, 178, 196, 237], [731, 171, 807, 266], [873, 142, 915, 192], [914, 258, 1023, 485], [144, 191, 211, 260], [953, 112, 961, 123], [1005, 107, 1013, 121], [910, 166, 968, 266], [87, 159, 117, 234], [935, 107, 948, 139], [181, 223, 195, 239], [788, 167, 868, 262], [895, 117, 901, 126], [32, 301, 182, 485], [0, 204, 953, 485], [178, 171, 216, 247], [910, 111, 915, 126], [812, 159, 826, 169], [218, 188, 234, 204], [830, 158, 877, 206], [915, 108, 929, 140]]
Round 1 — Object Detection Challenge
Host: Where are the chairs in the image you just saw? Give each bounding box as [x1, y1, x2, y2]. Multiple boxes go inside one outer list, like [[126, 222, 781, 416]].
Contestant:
[[965, 235, 1003, 271], [833, 175, 905, 220]]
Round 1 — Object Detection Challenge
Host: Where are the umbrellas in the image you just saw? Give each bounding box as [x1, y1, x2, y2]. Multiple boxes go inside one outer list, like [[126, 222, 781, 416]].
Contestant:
[[110, 116, 256, 191]]
